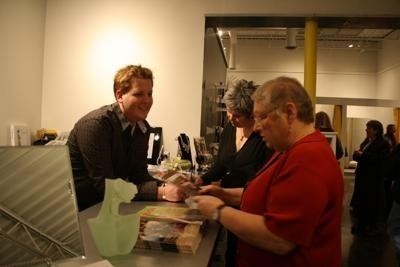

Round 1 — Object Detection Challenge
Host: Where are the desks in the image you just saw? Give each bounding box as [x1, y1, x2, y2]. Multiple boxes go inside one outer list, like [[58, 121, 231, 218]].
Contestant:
[[47, 198, 220, 266]]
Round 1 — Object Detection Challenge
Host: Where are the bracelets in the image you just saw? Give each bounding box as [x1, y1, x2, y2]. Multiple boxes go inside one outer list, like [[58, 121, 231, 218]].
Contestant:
[[162, 183, 166, 201]]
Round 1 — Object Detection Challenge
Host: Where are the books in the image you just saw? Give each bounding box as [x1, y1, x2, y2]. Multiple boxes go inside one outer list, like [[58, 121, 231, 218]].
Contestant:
[[134, 205, 209, 255]]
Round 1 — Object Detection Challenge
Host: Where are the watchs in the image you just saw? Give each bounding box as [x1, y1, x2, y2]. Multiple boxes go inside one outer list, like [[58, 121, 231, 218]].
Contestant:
[[211, 204, 228, 227]]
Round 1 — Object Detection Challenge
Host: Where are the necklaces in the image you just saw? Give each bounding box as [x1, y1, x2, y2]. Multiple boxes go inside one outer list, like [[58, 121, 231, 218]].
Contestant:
[[239, 131, 247, 141], [279, 129, 311, 153]]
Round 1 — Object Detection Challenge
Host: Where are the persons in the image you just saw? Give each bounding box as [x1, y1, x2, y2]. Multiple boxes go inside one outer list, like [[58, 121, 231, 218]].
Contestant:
[[66, 65, 190, 212], [350, 120, 400, 266], [315, 112, 344, 160], [193, 79, 275, 186], [184, 75, 344, 267]]
